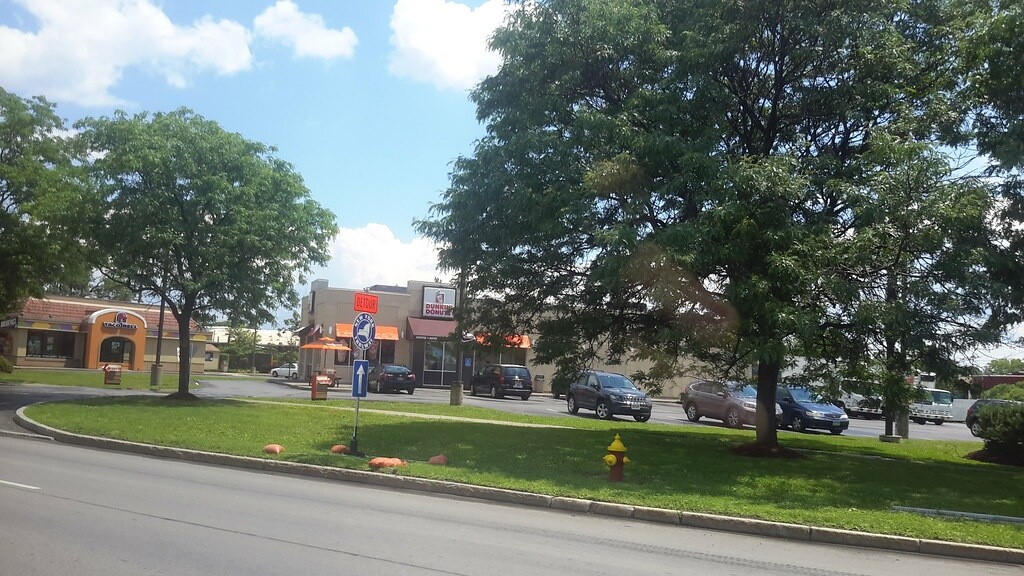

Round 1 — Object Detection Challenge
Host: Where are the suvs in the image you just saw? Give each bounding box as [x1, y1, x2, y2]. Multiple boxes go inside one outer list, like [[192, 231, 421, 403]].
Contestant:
[[965, 398, 1024, 438], [681, 379, 783, 432], [469, 364, 533, 401], [776, 382, 849, 437], [566, 370, 653, 422]]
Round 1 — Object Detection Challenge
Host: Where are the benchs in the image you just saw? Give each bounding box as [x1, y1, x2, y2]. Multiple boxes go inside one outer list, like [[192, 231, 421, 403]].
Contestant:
[[308, 376, 342, 387]]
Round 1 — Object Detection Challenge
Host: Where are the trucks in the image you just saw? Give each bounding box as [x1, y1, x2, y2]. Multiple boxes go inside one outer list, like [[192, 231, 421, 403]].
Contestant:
[[839, 376, 955, 425]]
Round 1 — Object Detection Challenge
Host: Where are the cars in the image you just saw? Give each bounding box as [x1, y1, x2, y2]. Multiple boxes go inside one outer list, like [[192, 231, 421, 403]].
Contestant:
[[368, 363, 407, 395], [271, 362, 299, 380]]
